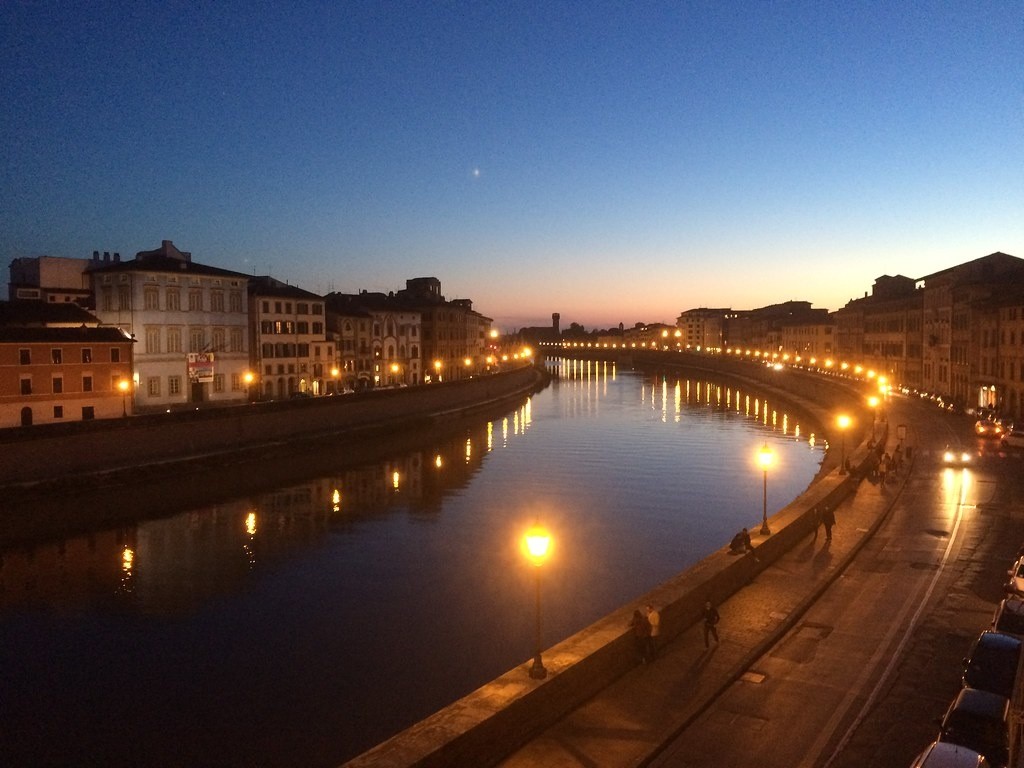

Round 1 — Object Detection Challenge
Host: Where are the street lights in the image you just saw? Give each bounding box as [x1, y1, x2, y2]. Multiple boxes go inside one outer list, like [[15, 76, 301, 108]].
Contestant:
[[870, 395, 877, 444], [117, 377, 133, 417], [241, 372, 256, 401], [836, 414, 849, 472], [245, 496, 257, 536], [330, 472, 341, 512], [435, 435, 443, 468], [756, 443, 772, 534], [330, 368, 339, 395], [467, 428, 472, 462], [487, 394, 532, 451], [663, 329, 668, 351], [119, 527, 134, 574], [435, 358, 441, 381], [541, 341, 890, 403], [465, 357, 470, 377], [520, 528, 556, 677], [391, 458, 400, 491], [390, 363, 400, 385], [674, 330, 681, 352], [545, 355, 828, 452], [486, 343, 531, 373]]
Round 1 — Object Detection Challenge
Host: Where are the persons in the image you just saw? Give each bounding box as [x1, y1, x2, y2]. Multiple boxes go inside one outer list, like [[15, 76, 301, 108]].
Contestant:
[[729, 528, 760, 564], [698, 602, 721, 651], [810, 505, 836, 541], [629, 604, 663, 664], [871, 445, 902, 487]]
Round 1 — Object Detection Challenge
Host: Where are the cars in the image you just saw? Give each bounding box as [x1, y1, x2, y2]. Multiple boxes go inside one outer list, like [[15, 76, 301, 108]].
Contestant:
[[975, 419, 1002, 435], [911, 554, 1024, 768], [1001, 430, 1024, 446], [894, 383, 1024, 430], [944, 442, 972, 464]]
